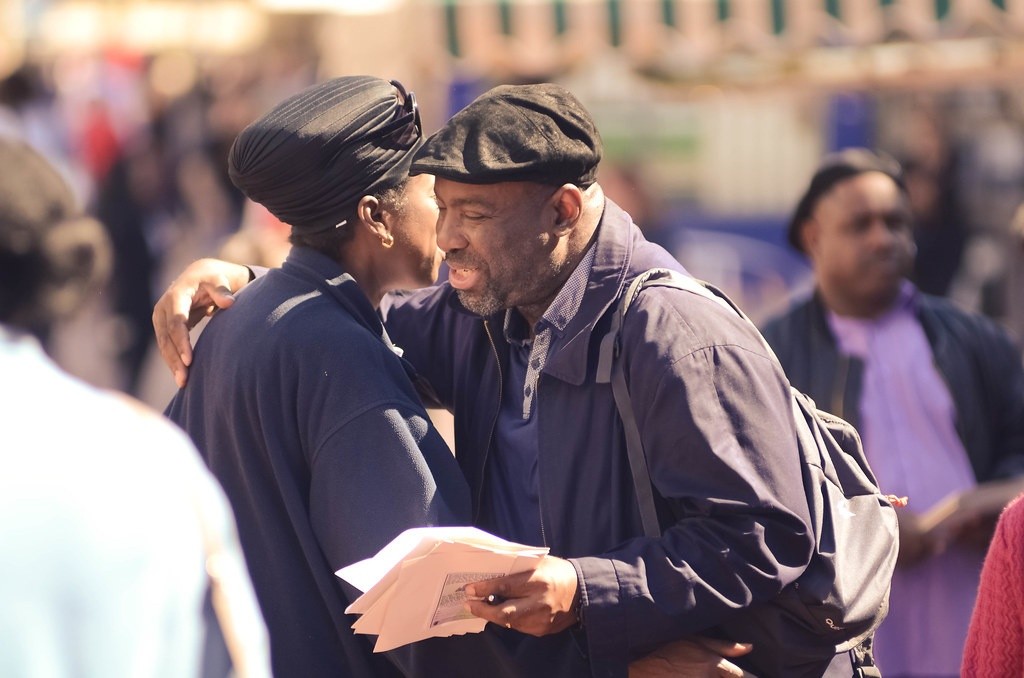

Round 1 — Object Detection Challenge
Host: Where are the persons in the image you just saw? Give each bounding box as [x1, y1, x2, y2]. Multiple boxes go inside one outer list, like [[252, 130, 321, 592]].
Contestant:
[[0, 61, 294, 408], [960, 492, 1024, 677], [161, 72, 753, 678], [0, 131, 272, 678], [150, 85, 883, 678], [753, 147, 1024, 678]]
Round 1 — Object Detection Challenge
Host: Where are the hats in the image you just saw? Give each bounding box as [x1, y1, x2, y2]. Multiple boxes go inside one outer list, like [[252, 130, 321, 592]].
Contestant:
[[228, 75, 427, 235], [787, 146, 912, 256], [409, 83, 604, 185]]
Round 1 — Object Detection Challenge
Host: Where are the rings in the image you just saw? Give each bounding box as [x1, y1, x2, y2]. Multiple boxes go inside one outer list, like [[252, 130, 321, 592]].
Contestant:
[[506, 622, 512, 629]]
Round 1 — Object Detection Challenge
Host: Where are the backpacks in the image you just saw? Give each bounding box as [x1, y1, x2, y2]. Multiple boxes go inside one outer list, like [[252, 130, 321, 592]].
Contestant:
[[596, 268, 908, 678]]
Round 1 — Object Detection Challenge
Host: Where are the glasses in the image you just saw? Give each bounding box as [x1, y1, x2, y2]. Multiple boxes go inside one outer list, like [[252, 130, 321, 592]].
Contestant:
[[325, 80, 422, 169]]
